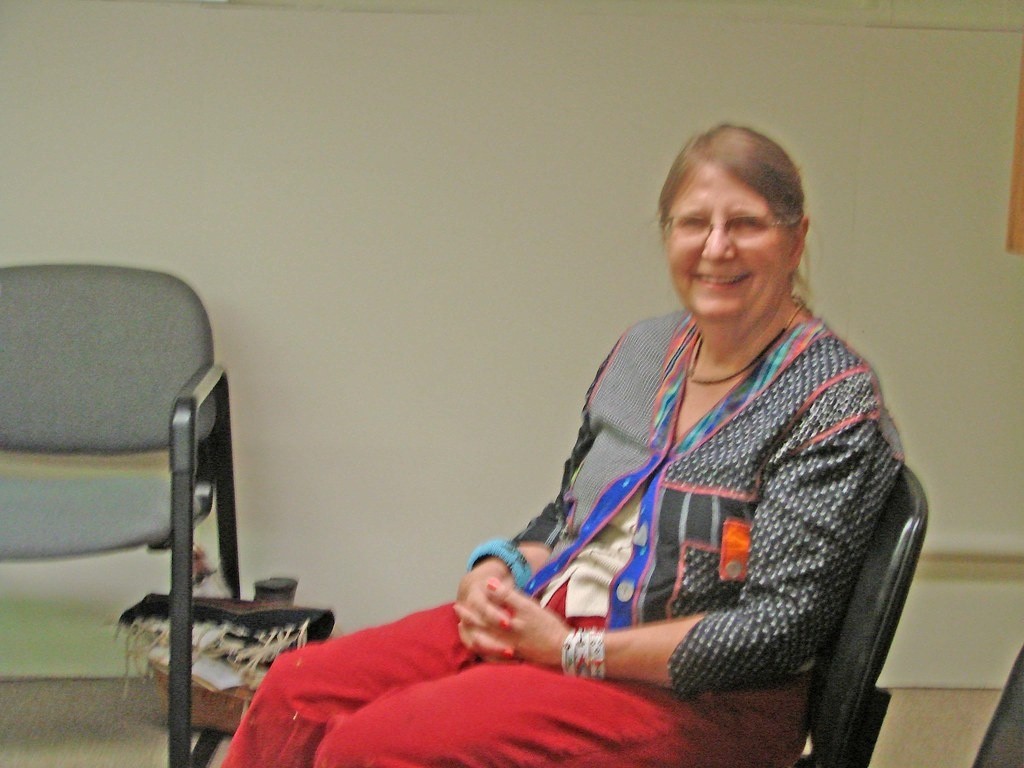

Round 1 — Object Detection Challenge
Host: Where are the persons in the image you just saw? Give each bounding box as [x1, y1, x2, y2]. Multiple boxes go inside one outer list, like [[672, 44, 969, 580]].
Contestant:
[[224, 126, 908, 768]]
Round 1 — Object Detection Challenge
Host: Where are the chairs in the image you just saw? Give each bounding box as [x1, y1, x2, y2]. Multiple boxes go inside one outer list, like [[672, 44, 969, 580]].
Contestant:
[[1, 264, 244, 768], [795, 462, 931, 768]]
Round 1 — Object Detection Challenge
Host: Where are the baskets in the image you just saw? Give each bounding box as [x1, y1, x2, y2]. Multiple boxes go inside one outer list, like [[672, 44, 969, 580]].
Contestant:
[[147, 663, 243, 735]]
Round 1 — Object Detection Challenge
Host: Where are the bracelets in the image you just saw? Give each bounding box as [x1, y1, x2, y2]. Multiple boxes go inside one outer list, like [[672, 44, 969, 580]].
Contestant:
[[467, 539, 532, 593], [563, 622, 606, 681]]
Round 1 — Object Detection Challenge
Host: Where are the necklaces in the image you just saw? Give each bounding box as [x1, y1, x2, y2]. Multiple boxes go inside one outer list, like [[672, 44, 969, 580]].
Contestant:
[[683, 293, 811, 388]]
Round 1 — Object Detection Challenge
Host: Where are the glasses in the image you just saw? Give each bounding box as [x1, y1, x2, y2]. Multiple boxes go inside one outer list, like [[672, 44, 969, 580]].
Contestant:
[[666, 211, 801, 241]]
